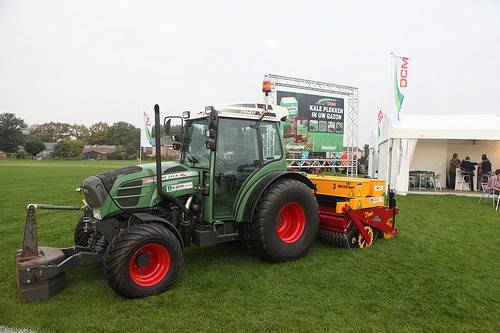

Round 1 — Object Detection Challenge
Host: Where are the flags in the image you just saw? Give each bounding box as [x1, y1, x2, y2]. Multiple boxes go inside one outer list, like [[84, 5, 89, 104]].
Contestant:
[[141, 108, 152, 147], [377, 108, 383, 137], [393, 55, 410, 121]]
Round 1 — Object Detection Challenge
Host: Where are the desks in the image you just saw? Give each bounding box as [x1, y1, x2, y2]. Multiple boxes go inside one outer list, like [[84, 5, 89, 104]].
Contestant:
[[419, 174, 430, 190]]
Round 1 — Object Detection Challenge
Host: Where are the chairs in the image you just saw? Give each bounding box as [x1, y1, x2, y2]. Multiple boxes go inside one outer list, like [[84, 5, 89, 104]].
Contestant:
[[476, 183, 493, 205], [429, 173, 443, 192], [409, 176, 415, 190]]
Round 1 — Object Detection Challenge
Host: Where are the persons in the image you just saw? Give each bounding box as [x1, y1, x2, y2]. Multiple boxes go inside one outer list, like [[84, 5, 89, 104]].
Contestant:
[[460, 156, 476, 191], [477, 157, 491, 191], [456, 159, 460, 164], [449, 153, 459, 189], [481, 154, 490, 162], [487, 169, 500, 206]]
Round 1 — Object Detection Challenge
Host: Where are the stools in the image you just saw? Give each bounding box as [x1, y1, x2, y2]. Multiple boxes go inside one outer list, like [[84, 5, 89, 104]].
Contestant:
[[455, 170, 471, 191]]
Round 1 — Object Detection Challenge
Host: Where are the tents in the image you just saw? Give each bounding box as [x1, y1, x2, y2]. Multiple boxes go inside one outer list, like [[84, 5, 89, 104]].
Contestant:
[[369, 115, 500, 196]]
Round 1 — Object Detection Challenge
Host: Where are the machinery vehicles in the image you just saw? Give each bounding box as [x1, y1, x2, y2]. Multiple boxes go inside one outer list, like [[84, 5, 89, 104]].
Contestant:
[[71, 77, 398, 299]]
